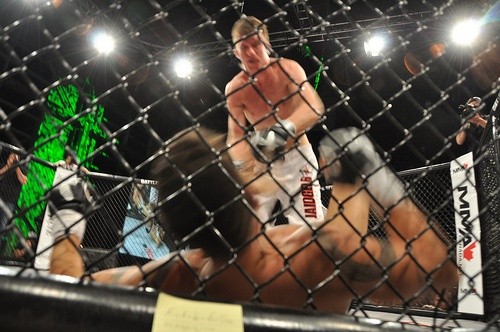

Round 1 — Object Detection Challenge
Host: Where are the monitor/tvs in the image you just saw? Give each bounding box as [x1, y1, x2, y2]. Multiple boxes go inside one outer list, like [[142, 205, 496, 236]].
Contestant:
[[117, 182, 171, 265]]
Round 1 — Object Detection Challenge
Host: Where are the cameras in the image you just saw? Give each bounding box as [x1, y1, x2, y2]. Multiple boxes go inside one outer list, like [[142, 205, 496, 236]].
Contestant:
[[457, 104, 475, 118]]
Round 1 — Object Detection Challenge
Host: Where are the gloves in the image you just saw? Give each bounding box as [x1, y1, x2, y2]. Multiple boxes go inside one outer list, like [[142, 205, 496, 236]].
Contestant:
[[248, 120, 296, 164], [45, 177, 102, 241], [319, 125, 376, 184]]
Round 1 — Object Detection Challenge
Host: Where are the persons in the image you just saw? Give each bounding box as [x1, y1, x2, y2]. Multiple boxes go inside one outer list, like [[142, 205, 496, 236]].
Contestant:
[[455, 97, 490, 154], [48, 128, 457, 316], [14, 232, 38, 261], [224, 16, 327, 231], [134, 184, 165, 247], [0, 152, 28, 241], [52, 155, 87, 176]]
